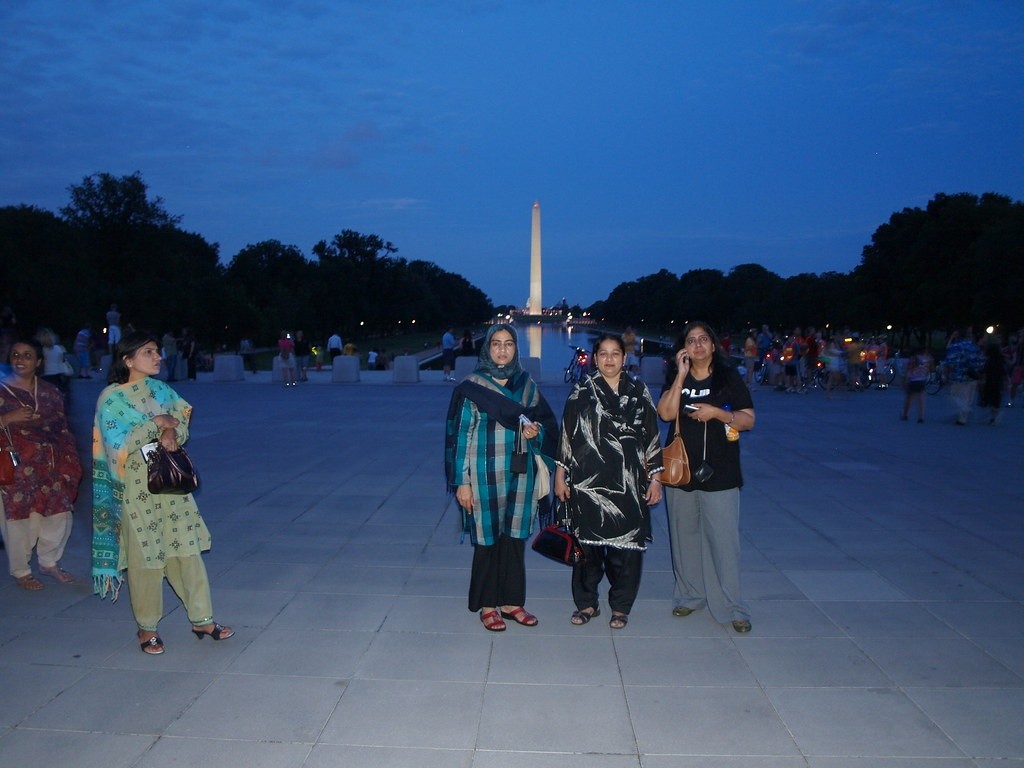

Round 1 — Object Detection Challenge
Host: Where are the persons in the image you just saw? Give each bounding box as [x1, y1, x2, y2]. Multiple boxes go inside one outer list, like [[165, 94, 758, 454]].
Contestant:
[[327, 331, 343, 364], [0, 337, 82, 591], [37, 325, 105, 402], [621, 327, 641, 375], [238, 335, 256, 373], [105, 304, 122, 354], [553, 333, 665, 628], [162, 329, 206, 383], [720, 324, 1024, 426], [657, 322, 755, 633], [344, 338, 360, 356], [314, 345, 324, 371], [367, 347, 391, 370], [460, 329, 475, 356], [441, 327, 457, 382], [89, 332, 235, 655], [277, 329, 312, 387], [444, 322, 560, 631]]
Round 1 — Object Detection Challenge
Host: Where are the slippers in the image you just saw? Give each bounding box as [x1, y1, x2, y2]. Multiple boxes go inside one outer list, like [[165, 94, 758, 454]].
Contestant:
[[38, 564, 73, 584], [501, 607, 539, 626], [15, 574, 44, 591], [480, 610, 507, 631]]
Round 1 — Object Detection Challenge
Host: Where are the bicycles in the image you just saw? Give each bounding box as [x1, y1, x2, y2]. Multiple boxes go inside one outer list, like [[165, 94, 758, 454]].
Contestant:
[[564, 346, 592, 384], [754, 347, 943, 394]]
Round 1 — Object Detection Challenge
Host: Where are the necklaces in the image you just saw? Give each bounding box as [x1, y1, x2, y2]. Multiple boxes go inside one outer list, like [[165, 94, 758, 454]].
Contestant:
[[0, 375, 39, 420]]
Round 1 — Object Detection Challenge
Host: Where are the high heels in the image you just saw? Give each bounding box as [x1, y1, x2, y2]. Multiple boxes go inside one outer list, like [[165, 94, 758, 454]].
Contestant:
[[138, 630, 164, 654], [192, 622, 236, 640]]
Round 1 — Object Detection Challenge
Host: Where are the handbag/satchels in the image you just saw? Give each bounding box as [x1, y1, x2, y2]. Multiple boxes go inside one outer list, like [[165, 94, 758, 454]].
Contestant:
[[63, 363, 74, 377], [0, 450, 14, 485], [644, 394, 691, 487], [147, 442, 197, 494], [531, 498, 586, 566]]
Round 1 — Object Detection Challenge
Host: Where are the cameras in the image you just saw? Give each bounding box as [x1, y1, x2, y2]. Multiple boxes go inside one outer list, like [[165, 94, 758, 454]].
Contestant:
[[519, 414, 532, 425], [286, 334, 291, 339], [8, 451, 22, 467], [682, 405, 700, 415]]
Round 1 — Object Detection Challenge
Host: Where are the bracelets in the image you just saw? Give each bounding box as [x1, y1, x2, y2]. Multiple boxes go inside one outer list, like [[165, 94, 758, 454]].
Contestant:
[[653, 479, 660, 483], [728, 411, 735, 424], [674, 381, 683, 388]]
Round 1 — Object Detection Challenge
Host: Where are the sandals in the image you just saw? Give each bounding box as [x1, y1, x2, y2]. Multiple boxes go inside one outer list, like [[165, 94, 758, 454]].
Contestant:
[[571, 604, 601, 625], [609, 612, 628, 629]]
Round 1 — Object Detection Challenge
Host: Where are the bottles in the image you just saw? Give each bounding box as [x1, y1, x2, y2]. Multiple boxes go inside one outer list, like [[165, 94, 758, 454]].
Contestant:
[[723, 404, 740, 441]]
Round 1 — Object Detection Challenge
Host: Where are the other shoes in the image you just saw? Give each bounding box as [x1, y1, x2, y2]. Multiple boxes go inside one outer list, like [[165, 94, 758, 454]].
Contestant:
[[918, 418, 923, 422], [672, 606, 695, 616], [732, 618, 752, 632], [957, 420, 965, 425], [900, 414, 908, 421], [79, 375, 92, 379]]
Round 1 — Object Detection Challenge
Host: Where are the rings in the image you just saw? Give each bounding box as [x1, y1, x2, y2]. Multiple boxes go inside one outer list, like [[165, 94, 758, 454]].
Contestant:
[[527, 432, 530, 435], [697, 417, 700, 422]]
